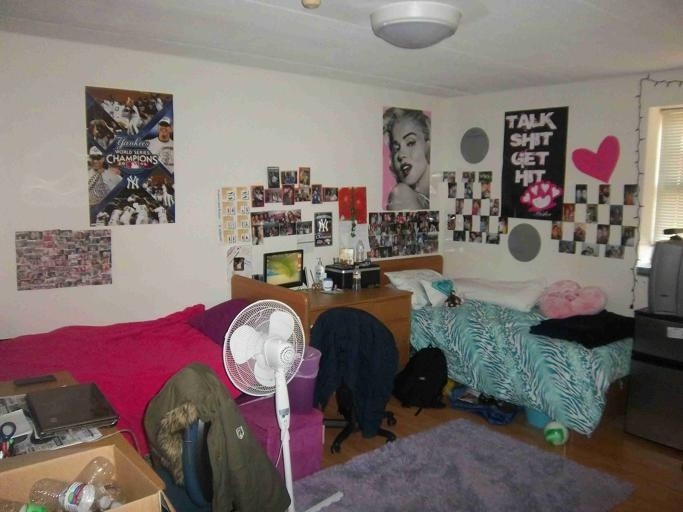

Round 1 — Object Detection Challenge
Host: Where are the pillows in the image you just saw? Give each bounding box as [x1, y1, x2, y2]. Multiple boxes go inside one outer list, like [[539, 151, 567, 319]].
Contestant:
[[383, 268, 606, 319], [188, 297, 252, 348]]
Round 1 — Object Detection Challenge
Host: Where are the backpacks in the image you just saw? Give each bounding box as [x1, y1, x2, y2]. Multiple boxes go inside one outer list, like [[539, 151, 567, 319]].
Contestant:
[[391, 344, 448, 416]]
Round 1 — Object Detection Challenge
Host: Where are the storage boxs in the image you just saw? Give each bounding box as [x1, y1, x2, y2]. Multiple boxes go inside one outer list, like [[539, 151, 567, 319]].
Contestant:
[[1, 428, 166, 511]]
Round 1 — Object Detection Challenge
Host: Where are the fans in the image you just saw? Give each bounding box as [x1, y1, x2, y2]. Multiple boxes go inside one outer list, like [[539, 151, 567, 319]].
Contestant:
[[223, 299, 343, 512]]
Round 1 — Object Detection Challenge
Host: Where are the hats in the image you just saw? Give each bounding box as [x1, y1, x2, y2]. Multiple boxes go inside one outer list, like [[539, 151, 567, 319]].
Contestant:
[[89, 146, 103, 157], [158, 116, 171, 124]]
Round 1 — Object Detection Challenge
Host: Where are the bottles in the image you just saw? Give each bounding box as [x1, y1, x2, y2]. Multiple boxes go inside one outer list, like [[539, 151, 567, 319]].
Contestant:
[[352, 265, 362, 291], [322, 278, 334, 292], [356, 239, 365, 262], [1, 456, 129, 511]]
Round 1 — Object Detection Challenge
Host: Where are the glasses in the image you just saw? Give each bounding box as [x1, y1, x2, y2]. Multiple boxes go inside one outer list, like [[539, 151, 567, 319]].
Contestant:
[[90, 156, 103, 160]]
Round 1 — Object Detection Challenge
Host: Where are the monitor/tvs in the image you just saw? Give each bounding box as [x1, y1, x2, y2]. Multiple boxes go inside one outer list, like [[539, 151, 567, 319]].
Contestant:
[[263, 249, 303, 288]]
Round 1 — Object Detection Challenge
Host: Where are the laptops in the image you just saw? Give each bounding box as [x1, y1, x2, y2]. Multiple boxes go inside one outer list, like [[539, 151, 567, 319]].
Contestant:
[[26, 383, 119, 437]]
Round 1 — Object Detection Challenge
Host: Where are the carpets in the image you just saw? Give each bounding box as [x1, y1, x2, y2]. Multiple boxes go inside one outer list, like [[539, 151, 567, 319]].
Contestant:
[[276, 417, 631, 511]]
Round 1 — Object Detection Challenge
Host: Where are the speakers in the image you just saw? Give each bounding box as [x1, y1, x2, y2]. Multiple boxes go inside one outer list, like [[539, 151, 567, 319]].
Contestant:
[[648, 240, 683, 317]]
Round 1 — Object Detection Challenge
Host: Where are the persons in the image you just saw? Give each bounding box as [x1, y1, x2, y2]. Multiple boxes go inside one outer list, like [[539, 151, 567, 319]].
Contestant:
[[381, 108, 431, 211], [84, 96, 173, 226]]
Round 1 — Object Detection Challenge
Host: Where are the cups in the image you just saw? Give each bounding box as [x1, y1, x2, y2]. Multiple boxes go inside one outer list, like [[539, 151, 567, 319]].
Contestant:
[[312, 283, 321, 293]]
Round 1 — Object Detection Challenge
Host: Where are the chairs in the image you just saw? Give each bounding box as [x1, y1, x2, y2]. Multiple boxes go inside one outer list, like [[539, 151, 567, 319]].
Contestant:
[[144, 361, 215, 512], [312, 304, 398, 452]]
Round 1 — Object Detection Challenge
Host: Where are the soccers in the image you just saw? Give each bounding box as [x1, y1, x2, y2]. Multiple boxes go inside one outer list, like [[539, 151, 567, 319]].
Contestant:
[[543, 421, 569, 446]]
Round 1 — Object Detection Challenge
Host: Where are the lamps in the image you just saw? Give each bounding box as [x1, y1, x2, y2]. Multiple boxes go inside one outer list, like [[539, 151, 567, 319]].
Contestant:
[[371, 1, 461, 51]]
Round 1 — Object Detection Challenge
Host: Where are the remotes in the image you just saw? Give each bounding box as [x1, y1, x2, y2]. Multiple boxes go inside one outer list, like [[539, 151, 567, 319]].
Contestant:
[[15, 375, 56, 385]]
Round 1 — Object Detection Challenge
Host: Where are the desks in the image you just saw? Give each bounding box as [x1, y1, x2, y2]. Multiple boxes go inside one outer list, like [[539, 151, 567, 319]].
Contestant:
[[283, 284, 414, 370], [1, 371, 166, 512]]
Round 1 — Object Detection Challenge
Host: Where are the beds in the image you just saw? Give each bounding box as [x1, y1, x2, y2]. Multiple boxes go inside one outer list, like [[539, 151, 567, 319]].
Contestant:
[[2, 276, 323, 481], [373, 255, 634, 435]]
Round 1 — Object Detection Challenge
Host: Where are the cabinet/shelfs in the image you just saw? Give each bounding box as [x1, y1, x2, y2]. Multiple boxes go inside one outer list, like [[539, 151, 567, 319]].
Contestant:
[[625, 308, 682, 453]]
